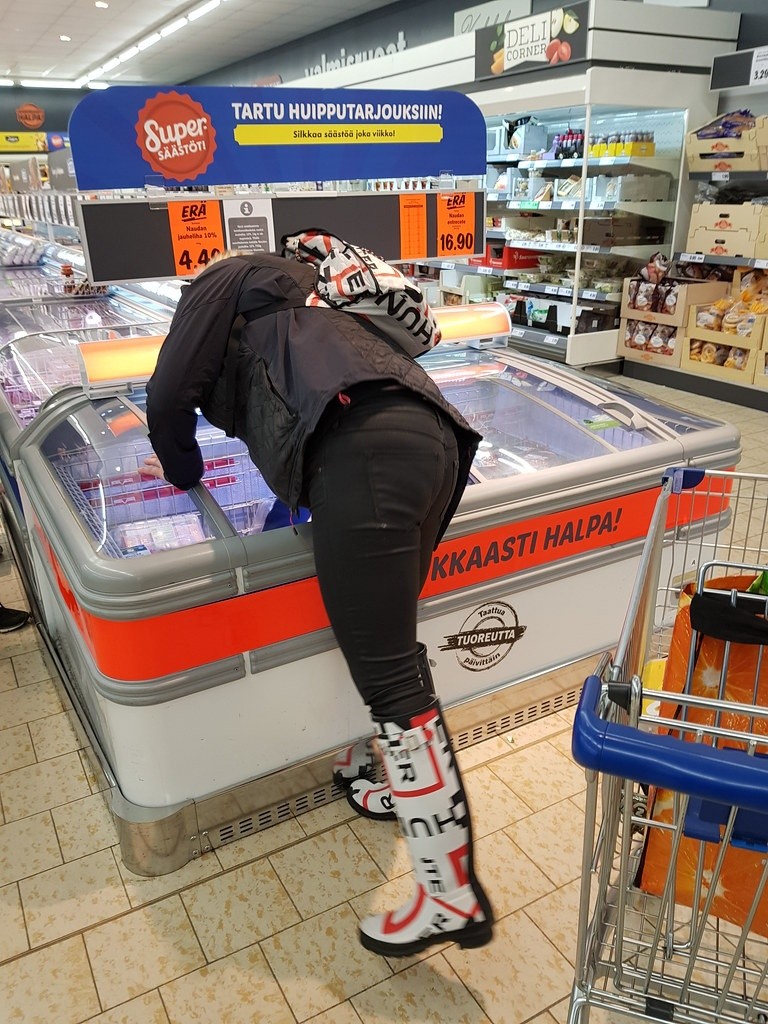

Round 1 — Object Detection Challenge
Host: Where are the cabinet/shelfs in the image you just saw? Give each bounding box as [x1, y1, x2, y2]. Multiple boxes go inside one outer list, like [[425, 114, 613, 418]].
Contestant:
[[622, 45, 768, 412], [409, 153, 741, 367]]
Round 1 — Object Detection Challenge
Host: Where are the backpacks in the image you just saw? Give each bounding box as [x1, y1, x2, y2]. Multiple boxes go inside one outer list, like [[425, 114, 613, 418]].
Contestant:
[[281, 227, 442, 358]]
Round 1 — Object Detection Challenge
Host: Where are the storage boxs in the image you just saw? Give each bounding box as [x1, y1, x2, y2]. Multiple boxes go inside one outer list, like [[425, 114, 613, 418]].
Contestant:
[[685, 203, 768, 260], [589, 141, 654, 156], [685, 114, 766, 172], [487, 245, 545, 268], [582, 218, 639, 245], [616, 269, 767, 392]]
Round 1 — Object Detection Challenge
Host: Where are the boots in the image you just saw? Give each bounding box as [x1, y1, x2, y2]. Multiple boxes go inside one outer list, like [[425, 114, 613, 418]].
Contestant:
[[332, 736, 380, 790], [356, 694, 493, 958], [346, 643, 433, 821]]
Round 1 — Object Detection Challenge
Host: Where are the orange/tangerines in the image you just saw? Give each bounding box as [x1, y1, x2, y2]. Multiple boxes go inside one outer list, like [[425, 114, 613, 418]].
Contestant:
[[639, 595, 768, 940]]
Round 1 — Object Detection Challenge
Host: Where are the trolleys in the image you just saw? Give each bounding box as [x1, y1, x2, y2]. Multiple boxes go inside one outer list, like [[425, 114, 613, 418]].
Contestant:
[[567, 462, 767, 1024]]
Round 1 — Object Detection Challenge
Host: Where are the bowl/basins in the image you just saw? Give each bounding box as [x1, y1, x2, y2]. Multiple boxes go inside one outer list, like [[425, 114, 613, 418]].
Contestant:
[[518, 250, 627, 294]]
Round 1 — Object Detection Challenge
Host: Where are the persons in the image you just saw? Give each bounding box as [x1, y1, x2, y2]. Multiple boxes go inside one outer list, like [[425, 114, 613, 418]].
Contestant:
[[0, 546, 29, 633], [145, 248, 496, 957]]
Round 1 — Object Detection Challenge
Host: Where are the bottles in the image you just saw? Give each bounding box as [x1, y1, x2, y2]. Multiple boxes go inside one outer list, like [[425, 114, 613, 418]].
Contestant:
[[551, 127, 655, 160], [60, 264, 75, 293]]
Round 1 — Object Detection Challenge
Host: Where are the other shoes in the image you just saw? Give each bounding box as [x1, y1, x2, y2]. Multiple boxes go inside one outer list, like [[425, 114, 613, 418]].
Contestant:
[[0, 603, 29, 633]]
